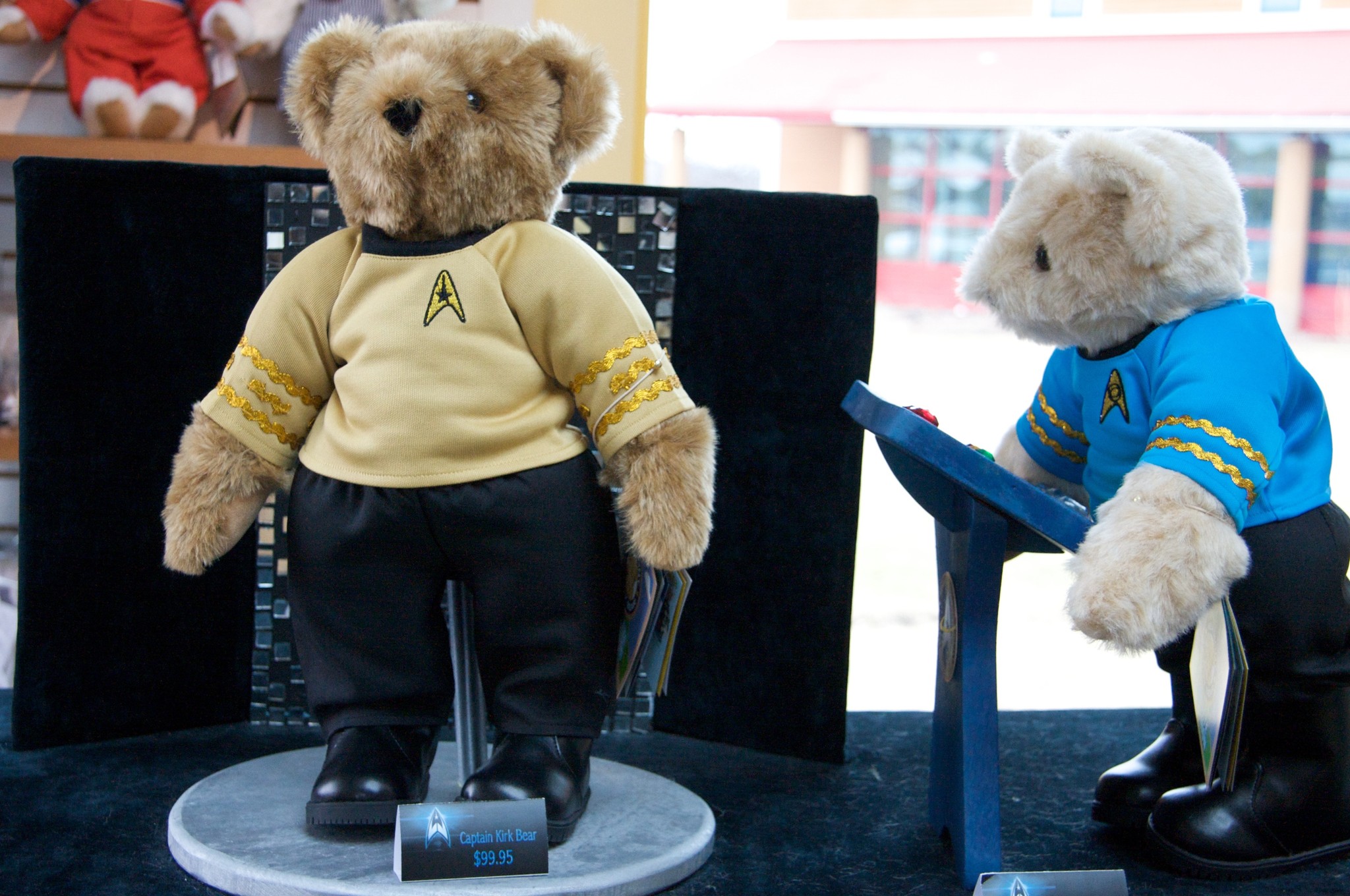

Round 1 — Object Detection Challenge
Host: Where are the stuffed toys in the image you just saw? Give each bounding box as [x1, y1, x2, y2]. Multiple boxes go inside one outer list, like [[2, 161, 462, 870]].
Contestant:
[[0, 0, 254, 142], [246, 0, 458, 147], [959, 126, 1350, 878], [161, 10, 716, 848]]
